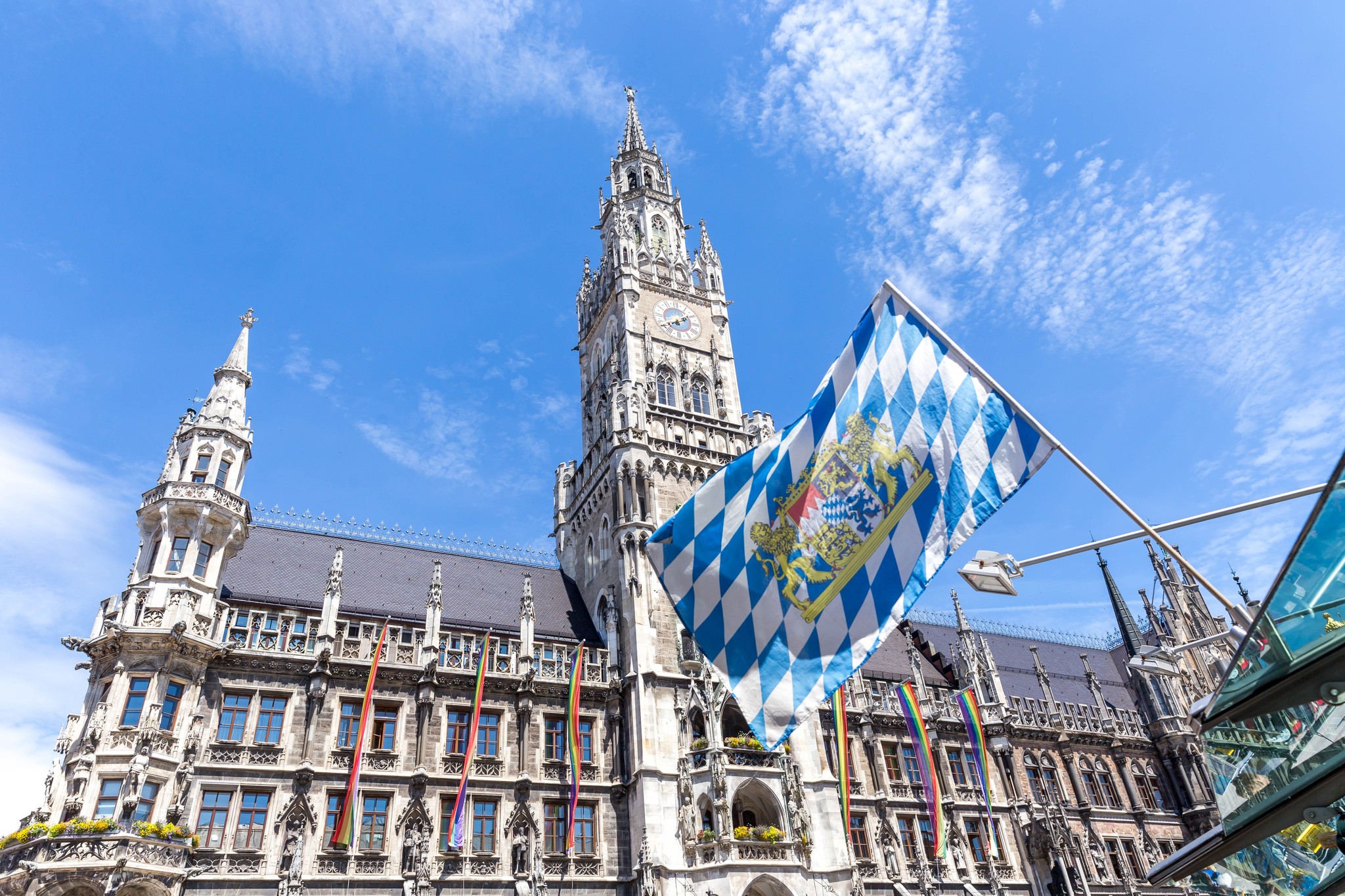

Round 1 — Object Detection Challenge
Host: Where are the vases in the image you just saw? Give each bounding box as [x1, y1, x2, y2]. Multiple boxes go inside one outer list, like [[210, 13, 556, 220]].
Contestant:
[[134, 829, 184, 843], [693, 744, 704, 750], [4, 840, 21, 848], [55, 829, 121, 835], [739, 836, 770, 841], [701, 837, 714, 843], [727, 743, 756, 749]]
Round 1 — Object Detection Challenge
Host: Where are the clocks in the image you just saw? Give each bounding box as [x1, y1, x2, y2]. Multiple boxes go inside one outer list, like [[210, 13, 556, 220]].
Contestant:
[[653, 299, 702, 340]]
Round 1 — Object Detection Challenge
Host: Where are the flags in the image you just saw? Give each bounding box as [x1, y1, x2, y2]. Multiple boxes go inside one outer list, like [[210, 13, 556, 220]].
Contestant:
[[566, 644, 584, 850], [448, 633, 489, 847], [332, 621, 389, 849], [643, 281, 1062, 753]]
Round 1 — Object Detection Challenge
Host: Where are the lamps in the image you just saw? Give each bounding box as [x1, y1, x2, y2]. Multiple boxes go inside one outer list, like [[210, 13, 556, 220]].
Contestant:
[[957, 480, 1345, 595], [1124, 624, 1261, 678]]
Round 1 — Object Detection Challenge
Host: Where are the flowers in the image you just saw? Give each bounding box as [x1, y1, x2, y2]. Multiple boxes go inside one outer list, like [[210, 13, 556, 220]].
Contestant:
[[803, 835, 808, 846], [0, 822, 202, 850], [723, 730, 765, 751], [783, 740, 794, 754], [689, 735, 711, 752], [734, 822, 787, 846], [697, 827, 715, 842]]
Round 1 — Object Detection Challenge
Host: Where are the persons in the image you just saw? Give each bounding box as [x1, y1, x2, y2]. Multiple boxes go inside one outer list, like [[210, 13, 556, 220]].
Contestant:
[[1146, 847, 1157, 867], [1049, 860, 1067, 893], [513, 827, 530, 875], [798, 801, 814, 845], [952, 838, 968, 877], [43, 755, 62, 808], [281, 821, 301, 872], [124, 745, 150, 798], [883, 839, 900, 879], [713, 790, 731, 837], [403, 822, 422, 873], [171, 753, 196, 806], [788, 795, 805, 839], [65, 742, 95, 800], [677, 796, 696, 841], [1090, 843, 1112, 880]]
